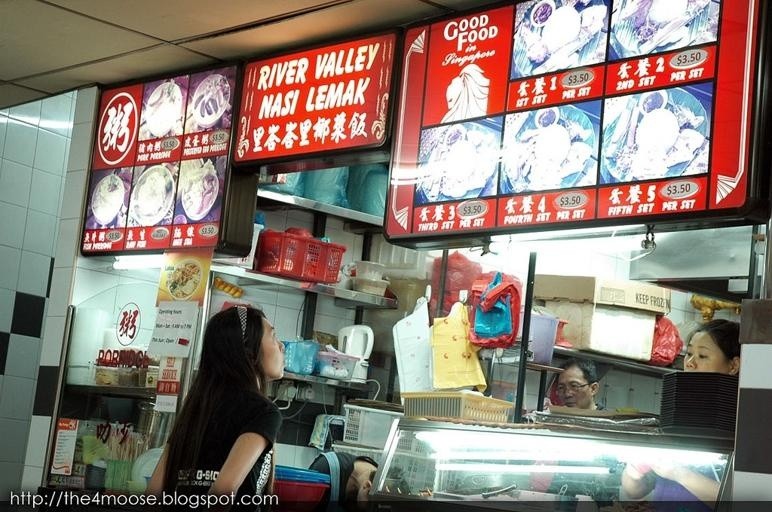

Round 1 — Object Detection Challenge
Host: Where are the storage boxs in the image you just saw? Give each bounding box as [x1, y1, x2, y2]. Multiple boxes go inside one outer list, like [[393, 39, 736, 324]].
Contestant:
[[535, 272, 673, 362]]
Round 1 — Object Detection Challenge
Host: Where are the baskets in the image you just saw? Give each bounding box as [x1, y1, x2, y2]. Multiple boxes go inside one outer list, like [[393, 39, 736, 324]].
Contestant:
[[400, 391, 515, 423]]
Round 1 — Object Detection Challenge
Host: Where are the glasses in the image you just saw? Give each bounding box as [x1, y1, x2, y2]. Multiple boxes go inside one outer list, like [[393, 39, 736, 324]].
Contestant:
[[553, 382, 591, 395]]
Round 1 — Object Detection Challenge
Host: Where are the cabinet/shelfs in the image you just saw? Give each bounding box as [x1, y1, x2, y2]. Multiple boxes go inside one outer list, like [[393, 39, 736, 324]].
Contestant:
[[172, 186, 401, 403]]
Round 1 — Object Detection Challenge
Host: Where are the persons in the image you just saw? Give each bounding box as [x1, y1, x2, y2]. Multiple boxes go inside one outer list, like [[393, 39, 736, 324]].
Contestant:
[[547, 356, 615, 506], [142, 306, 287, 511], [311, 451, 411, 512], [620, 318, 739, 510]]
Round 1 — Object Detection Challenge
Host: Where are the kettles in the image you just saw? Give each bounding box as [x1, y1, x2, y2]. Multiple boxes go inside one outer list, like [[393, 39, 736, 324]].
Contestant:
[[336, 326, 374, 385]]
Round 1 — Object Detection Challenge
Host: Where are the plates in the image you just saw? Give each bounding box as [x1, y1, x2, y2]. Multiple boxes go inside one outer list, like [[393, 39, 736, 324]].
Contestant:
[[91, 75, 230, 226], [168, 259, 203, 300], [131, 449, 164, 481]]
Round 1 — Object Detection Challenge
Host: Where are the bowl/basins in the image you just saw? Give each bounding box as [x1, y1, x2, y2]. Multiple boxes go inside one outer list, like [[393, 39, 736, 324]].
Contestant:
[[659, 370, 739, 453], [638, 87, 668, 115], [534, 106, 560, 127], [273, 463, 331, 500], [529, 0, 556, 28], [351, 257, 390, 296]]
